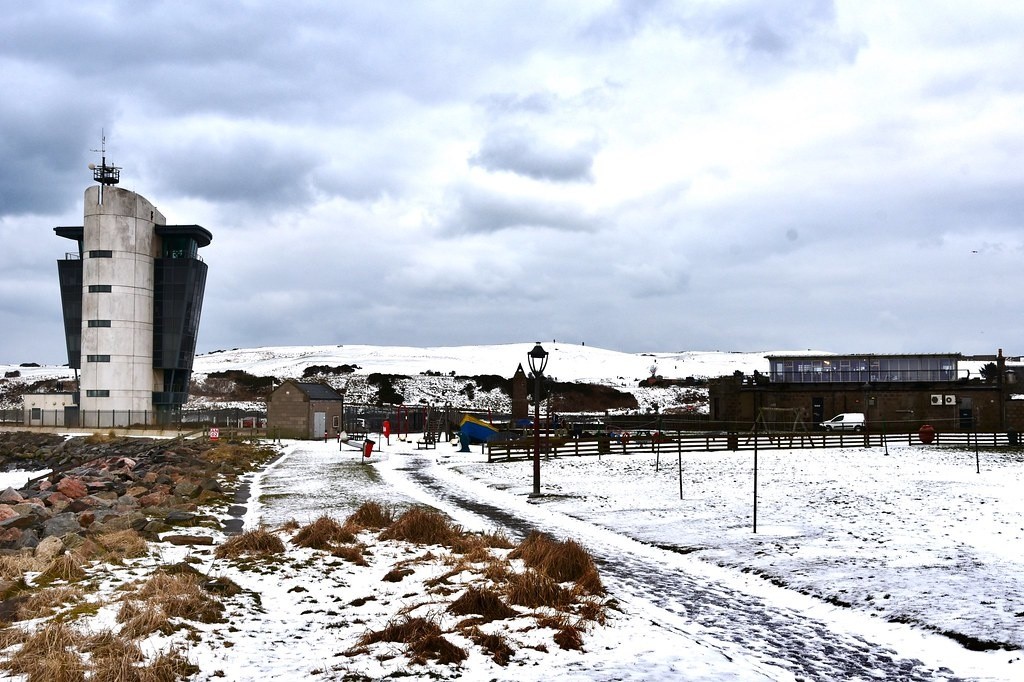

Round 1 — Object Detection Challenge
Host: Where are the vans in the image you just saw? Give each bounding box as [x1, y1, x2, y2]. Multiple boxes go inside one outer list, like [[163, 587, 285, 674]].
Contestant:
[[819, 413, 866, 431]]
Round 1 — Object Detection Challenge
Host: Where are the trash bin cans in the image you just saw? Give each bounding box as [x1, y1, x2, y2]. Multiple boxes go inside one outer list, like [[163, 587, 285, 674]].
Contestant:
[[365, 439, 375, 457]]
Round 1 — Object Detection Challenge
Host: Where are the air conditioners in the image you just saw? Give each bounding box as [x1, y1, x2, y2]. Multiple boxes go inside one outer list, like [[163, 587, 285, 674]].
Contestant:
[[931, 394, 942, 405], [945, 394, 957, 406]]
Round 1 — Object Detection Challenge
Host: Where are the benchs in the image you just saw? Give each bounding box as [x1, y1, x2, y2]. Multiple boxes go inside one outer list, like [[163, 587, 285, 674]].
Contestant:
[[347, 432, 365, 441]]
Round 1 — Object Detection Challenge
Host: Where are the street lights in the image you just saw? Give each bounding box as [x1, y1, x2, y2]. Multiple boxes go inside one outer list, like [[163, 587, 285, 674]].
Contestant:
[[528, 340, 550, 500]]
